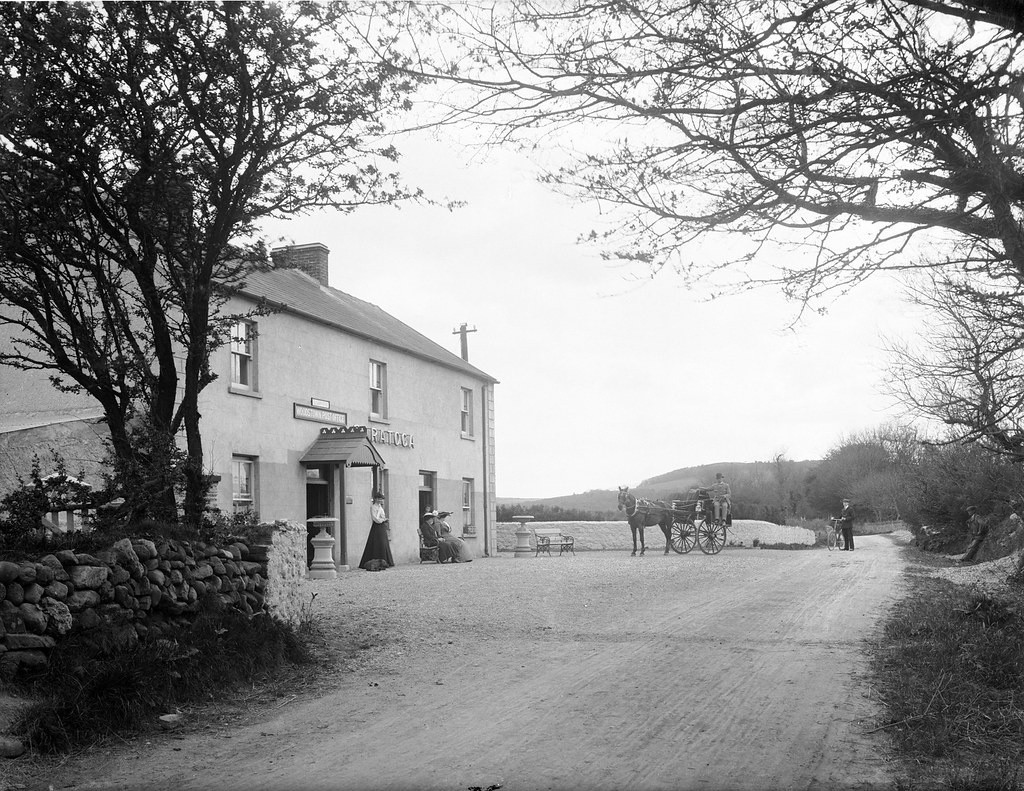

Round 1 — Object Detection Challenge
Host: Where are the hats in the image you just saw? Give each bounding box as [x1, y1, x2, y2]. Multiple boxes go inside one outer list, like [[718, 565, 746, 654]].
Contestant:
[[715, 473, 724, 479], [843, 499, 850, 502]]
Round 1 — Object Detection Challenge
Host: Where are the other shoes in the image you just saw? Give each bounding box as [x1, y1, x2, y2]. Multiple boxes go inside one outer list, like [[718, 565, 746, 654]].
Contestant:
[[840, 549, 848, 551], [715, 519, 719, 525], [850, 549, 853, 551], [722, 520, 726, 526]]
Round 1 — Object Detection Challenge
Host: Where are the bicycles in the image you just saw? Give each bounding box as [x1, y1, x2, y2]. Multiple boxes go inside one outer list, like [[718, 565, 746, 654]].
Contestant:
[[827, 518, 848, 551]]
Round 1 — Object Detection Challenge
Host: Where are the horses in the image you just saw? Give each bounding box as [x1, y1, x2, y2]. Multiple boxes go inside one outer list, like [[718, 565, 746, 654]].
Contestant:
[[619, 487, 675, 556]]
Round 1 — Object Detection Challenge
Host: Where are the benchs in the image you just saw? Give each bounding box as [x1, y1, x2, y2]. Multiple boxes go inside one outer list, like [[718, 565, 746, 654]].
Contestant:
[[534, 528, 576, 557], [417, 528, 465, 564]]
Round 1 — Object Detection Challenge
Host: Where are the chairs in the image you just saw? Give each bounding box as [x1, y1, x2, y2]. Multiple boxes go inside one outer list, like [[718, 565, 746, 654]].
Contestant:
[[673, 489, 699, 513]]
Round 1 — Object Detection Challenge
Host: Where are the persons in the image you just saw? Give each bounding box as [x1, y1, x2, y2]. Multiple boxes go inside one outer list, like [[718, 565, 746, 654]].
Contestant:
[[830, 499, 855, 551], [420, 505, 472, 562], [696, 472, 732, 526], [357, 491, 395, 573]]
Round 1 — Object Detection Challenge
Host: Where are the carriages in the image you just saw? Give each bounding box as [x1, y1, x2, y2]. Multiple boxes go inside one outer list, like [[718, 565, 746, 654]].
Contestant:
[[617, 486, 733, 555]]
